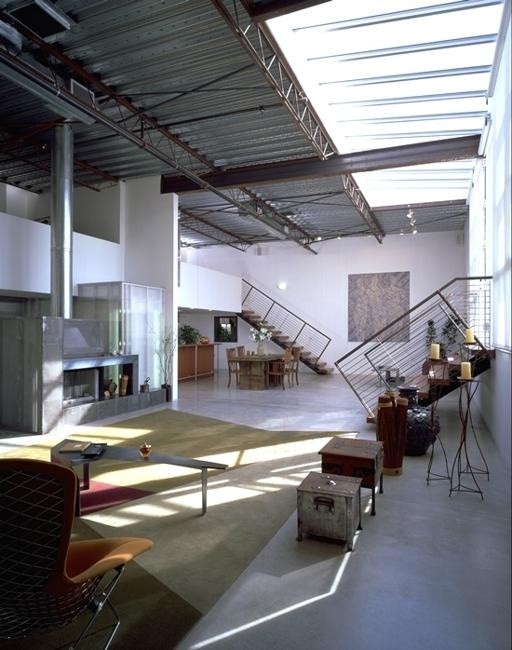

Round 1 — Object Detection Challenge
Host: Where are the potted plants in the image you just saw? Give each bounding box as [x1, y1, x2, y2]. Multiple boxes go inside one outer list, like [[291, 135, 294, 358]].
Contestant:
[[422, 316, 468, 374], [179, 321, 200, 344]]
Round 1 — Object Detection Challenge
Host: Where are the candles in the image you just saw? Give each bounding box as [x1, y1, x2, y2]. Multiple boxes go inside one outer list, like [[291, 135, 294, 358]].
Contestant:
[[461, 361, 472, 379], [465, 328, 474, 343], [430, 344, 440, 359]]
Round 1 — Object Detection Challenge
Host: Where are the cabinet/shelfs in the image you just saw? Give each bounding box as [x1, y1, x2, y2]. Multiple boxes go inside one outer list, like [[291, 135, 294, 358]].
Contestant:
[[295, 471, 362, 551], [449, 377, 489, 499], [456, 341, 488, 480], [319, 437, 383, 516], [178, 344, 214, 383], [425, 360, 451, 488]]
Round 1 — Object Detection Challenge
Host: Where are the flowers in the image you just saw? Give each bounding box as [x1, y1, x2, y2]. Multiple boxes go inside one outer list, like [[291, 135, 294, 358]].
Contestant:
[[248, 322, 272, 343]]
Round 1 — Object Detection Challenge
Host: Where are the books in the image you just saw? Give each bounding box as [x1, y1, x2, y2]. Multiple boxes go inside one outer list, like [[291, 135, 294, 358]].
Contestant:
[[58, 440, 92, 452]]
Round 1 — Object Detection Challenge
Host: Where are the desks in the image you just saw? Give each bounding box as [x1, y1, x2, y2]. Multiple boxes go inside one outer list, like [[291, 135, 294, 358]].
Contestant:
[[228, 355, 287, 390]]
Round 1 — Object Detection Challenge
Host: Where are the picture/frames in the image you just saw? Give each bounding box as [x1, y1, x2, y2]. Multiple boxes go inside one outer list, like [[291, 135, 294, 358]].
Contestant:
[[256, 341, 264, 354]]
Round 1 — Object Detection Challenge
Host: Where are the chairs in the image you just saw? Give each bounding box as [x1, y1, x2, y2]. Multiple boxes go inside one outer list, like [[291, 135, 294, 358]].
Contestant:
[[237, 346, 245, 357], [265, 348, 292, 391], [225, 348, 241, 387], [0, 459, 154, 650], [281, 347, 304, 386]]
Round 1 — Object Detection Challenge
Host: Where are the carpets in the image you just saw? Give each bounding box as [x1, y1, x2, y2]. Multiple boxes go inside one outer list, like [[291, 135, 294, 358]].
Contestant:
[[1, 408, 358, 650], [77, 477, 154, 514]]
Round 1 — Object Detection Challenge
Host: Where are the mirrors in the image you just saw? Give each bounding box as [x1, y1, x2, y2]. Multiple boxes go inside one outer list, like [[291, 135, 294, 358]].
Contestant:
[[214, 315, 238, 342]]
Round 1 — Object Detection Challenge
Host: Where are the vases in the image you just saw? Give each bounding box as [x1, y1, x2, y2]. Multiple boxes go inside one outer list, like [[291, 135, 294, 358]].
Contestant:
[[161, 385, 171, 402]]
